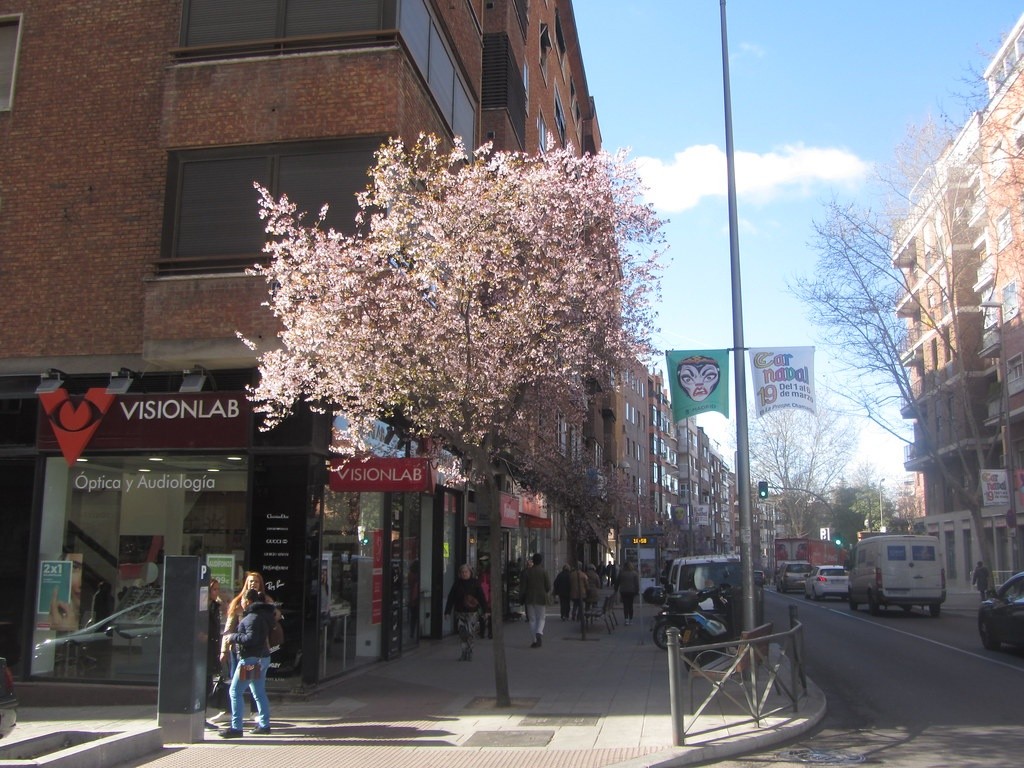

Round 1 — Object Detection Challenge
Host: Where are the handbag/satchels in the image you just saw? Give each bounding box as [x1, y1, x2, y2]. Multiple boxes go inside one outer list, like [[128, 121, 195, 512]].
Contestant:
[[616, 592, 621, 606], [240, 663, 260, 680], [210, 672, 226, 708], [269, 623, 284, 647], [547, 593, 554, 607], [464, 595, 479, 608]]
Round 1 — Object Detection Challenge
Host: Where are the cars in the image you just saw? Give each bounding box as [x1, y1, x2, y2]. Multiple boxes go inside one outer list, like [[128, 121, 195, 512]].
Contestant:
[[31, 597, 163, 686], [0, 656, 18, 743], [322, 554, 374, 632], [803, 565, 849, 602], [978, 572, 1024, 651]]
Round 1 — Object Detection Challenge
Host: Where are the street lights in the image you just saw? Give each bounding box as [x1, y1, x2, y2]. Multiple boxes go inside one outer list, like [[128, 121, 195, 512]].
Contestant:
[[880, 478, 886, 526]]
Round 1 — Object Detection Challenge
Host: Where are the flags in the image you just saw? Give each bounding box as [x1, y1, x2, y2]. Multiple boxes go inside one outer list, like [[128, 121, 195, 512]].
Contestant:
[[749, 346, 817, 419], [665, 349, 729, 424]]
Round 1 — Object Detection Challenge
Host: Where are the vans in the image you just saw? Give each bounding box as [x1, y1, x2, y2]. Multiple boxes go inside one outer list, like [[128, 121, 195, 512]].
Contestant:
[[844, 534, 947, 617], [775, 560, 812, 593]]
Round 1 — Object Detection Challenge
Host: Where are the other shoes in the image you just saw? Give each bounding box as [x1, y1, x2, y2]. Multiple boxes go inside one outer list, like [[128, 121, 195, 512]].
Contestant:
[[625, 618, 633, 626], [531, 633, 542, 647]]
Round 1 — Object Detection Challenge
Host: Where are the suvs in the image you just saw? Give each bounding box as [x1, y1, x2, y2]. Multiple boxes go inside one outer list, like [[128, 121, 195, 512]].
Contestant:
[[754, 571, 765, 587]]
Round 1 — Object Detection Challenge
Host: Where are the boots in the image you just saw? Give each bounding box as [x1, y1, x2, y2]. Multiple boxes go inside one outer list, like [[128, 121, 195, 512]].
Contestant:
[[457, 648, 473, 662]]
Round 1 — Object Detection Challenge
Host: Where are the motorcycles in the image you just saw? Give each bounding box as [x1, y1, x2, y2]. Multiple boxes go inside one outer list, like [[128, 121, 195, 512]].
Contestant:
[[650, 583, 733, 671]]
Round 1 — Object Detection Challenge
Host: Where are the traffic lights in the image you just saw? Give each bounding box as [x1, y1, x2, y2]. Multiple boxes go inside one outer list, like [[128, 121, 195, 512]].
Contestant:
[[759, 481, 768, 499], [834, 532, 842, 547]]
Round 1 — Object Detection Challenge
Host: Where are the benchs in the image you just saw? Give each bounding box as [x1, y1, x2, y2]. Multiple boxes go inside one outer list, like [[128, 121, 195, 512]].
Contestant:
[[584, 592, 618, 635]]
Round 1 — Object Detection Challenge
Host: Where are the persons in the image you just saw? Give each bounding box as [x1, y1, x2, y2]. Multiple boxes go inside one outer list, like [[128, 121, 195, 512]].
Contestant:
[[205, 579, 221, 730], [443, 564, 489, 662], [519, 553, 551, 648], [210, 572, 284, 723], [91, 582, 114, 672], [335, 560, 419, 644], [478, 564, 493, 639], [594, 561, 616, 588], [615, 560, 639, 625], [972, 561, 990, 601], [49, 553, 83, 632], [554, 560, 600, 623], [219, 589, 274, 738]]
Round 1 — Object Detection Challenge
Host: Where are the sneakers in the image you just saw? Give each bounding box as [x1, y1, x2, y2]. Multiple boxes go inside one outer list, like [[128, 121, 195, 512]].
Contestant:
[[219, 728, 243, 738], [204, 721, 219, 731], [249, 726, 271, 734], [211, 713, 232, 722], [250, 712, 260, 723]]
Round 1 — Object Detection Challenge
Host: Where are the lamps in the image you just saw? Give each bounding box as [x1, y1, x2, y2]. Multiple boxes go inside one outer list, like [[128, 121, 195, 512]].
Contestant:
[[35, 368, 71, 394], [104, 367, 145, 393], [179, 363, 219, 394]]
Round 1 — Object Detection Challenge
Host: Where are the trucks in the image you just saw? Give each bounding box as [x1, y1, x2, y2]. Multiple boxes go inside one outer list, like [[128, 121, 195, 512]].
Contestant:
[[774, 538, 850, 574]]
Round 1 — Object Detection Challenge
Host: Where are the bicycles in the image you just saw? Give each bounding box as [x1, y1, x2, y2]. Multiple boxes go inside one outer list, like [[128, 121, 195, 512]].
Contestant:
[[63, 625, 149, 682]]
